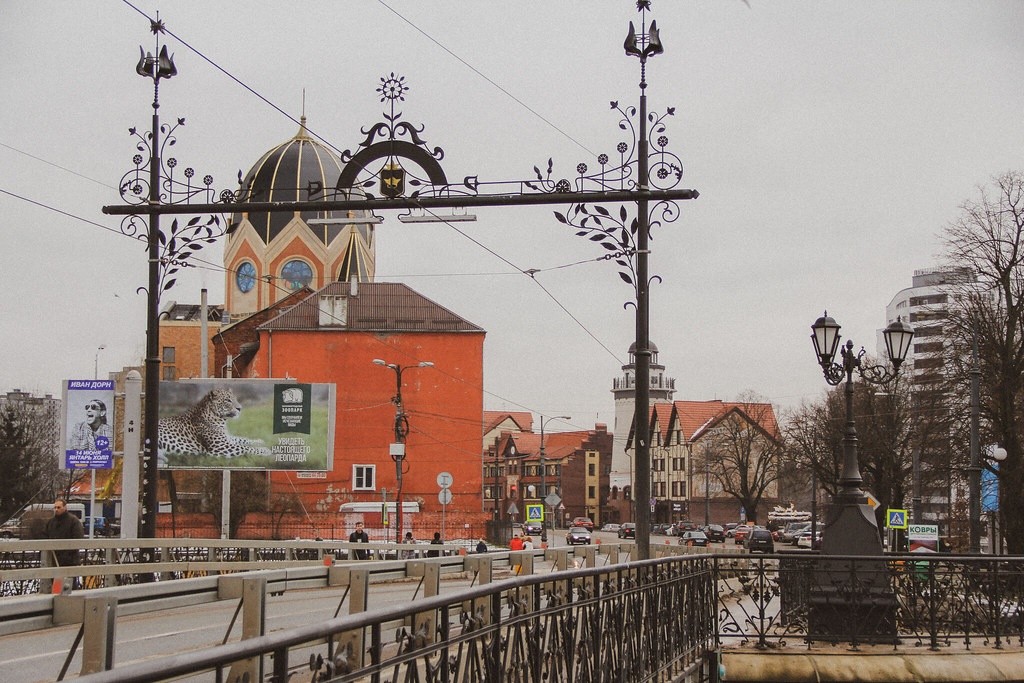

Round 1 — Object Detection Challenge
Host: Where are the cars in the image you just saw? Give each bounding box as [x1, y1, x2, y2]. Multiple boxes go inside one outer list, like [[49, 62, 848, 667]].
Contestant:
[[565, 527, 592, 545], [618, 522, 636, 539], [570, 516, 594, 532], [772, 520, 825, 548], [650, 521, 766, 545], [84, 516, 107, 535], [678, 531, 710, 547], [522, 521, 543, 535], [109, 524, 121, 535], [747, 529, 775, 553]]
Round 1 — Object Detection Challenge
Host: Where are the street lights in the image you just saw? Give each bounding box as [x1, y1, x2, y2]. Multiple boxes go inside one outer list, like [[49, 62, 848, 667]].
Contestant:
[[372, 357, 441, 560], [801, 305, 916, 643], [89, 343, 108, 539], [994, 447, 1008, 556], [540, 415, 572, 547]]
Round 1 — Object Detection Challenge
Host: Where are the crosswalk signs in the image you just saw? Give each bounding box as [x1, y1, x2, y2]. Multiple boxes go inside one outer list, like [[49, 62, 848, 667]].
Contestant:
[[527, 505, 543, 522], [886, 509, 907, 529]]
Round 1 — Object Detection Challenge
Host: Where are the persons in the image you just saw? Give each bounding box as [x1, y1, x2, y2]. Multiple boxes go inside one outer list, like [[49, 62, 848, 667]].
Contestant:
[[40, 499, 83, 567], [401, 532, 416, 559], [476, 540, 487, 554], [71, 399, 113, 451], [349, 522, 370, 560], [508, 533, 533, 550], [427, 532, 443, 557]]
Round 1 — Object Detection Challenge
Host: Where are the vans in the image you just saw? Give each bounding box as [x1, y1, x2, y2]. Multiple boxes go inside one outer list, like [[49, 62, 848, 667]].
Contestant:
[[0, 503, 89, 540], [605, 524, 621, 533]]
